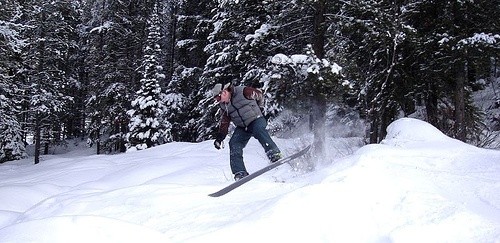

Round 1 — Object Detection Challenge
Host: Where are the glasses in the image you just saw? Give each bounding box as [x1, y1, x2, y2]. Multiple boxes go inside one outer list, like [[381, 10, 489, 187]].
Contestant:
[[214, 95, 221, 103]]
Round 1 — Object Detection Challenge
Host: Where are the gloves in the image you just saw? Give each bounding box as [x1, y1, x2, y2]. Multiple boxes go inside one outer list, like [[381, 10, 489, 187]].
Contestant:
[[214, 135, 224, 149], [253, 90, 263, 100]]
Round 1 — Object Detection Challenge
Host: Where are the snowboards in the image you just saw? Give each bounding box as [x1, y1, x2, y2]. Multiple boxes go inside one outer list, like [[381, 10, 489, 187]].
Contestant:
[[208, 144, 311, 197]]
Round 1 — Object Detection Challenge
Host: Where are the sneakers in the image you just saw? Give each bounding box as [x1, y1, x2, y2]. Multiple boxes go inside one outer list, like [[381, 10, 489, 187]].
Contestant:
[[271, 151, 282, 162], [234, 170, 249, 181]]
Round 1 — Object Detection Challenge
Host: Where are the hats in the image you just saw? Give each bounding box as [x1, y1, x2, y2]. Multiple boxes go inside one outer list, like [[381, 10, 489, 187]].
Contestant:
[[212, 82, 230, 98]]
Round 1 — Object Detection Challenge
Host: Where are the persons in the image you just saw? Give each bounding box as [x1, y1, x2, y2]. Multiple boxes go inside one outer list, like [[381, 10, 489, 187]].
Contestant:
[[211, 83, 284, 182]]
